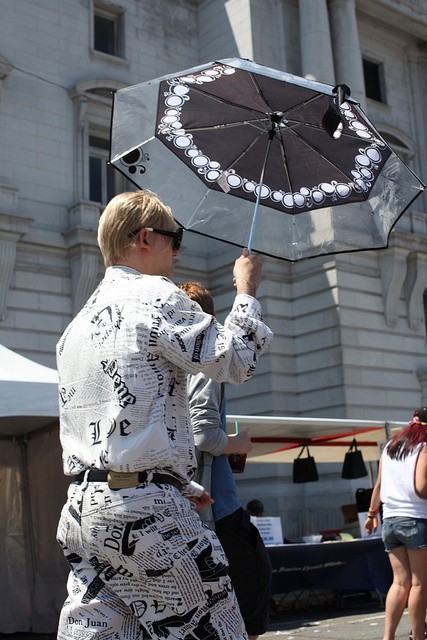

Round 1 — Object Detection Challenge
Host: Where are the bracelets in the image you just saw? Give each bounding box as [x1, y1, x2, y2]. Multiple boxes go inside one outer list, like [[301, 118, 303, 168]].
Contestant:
[[367, 506, 378, 534], [233, 277, 259, 292]]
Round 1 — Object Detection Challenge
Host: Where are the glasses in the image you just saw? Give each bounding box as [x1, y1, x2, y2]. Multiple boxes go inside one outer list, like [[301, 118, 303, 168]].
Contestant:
[[128, 226, 184, 251]]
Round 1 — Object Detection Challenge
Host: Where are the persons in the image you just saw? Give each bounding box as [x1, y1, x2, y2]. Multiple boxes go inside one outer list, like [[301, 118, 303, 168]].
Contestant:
[[247, 499, 265, 516], [174, 281, 272, 640], [55, 186, 273, 639], [364, 406, 427, 640]]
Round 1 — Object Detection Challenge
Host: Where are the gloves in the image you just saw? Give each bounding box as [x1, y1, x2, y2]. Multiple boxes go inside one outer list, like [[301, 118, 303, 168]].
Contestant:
[[356, 487, 374, 512]]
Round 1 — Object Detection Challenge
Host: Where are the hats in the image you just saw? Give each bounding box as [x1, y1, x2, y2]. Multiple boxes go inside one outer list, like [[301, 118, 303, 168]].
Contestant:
[[413, 406, 427, 427]]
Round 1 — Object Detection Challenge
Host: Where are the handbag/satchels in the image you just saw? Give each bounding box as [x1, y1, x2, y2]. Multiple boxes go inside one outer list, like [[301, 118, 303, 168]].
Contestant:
[[341, 438, 369, 479], [293, 444, 320, 484]]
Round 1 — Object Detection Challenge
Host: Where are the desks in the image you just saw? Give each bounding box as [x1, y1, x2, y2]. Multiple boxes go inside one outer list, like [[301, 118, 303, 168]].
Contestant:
[[258, 535, 393, 610]]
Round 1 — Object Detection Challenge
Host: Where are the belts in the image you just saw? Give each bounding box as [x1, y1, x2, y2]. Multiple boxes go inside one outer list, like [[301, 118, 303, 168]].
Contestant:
[[73, 466, 184, 494]]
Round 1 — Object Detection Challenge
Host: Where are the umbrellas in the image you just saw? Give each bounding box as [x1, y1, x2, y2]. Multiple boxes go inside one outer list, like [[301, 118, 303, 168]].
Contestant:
[[106, 57, 426, 264]]
